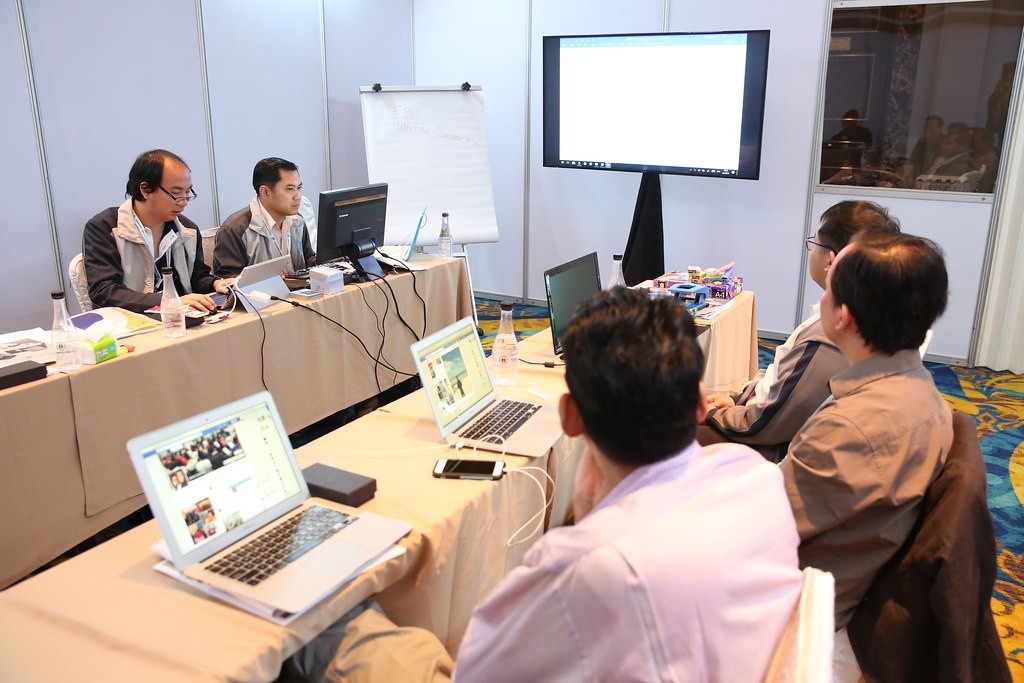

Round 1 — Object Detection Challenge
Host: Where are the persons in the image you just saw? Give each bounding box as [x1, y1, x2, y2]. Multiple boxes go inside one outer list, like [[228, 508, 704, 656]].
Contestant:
[[163, 429, 239, 541], [698, 200, 900, 461], [429, 366, 465, 398], [213, 157, 316, 278], [82, 149, 236, 313], [290, 284, 804, 683], [780, 232, 954, 635], [834, 63, 1014, 192]]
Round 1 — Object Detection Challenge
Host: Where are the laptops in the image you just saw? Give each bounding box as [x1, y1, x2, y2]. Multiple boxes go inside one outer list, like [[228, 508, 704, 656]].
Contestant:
[[126, 390, 412, 615], [374, 205, 427, 261], [410, 315, 564, 458], [544, 252, 602, 360], [208, 254, 292, 311]]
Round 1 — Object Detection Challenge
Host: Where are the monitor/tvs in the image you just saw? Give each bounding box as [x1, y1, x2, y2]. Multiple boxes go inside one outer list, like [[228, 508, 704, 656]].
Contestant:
[[315, 182, 387, 283], [542, 30, 770, 181]]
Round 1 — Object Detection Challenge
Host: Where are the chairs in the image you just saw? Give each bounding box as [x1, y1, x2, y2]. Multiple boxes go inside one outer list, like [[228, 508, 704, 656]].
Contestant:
[[200, 226, 219, 276], [832, 412, 1014, 683], [67, 253, 94, 313], [762, 566, 837, 683]]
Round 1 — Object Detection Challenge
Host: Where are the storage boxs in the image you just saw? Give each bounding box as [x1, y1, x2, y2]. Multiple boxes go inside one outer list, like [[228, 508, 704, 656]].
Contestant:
[[706, 276, 744, 300], [654, 272, 688, 289], [0, 360, 48, 391], [185, 316, 205, 329], [302, 462, 377, 510]]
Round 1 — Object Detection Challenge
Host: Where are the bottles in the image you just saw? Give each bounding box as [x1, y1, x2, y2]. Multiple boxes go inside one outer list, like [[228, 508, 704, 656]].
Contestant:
[[438, 212, 452, 260], [492, 300, 518, 387], [607, 255, 626, 291], [50, 291, 82, 372], [161, 266, 186, 338]]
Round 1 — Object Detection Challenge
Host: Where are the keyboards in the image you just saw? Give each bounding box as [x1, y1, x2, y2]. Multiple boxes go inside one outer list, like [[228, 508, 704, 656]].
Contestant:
[[284, 261, 348, 280]]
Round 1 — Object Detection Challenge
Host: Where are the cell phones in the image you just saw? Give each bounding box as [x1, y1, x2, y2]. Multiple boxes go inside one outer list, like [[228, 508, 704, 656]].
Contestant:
[[432, 459, 506, 480]]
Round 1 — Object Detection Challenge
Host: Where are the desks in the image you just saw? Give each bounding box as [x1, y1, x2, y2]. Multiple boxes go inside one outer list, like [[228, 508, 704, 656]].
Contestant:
[[0, 252, 472, 592], [0, 407, 552, 683], [383, 279, 761, 531]]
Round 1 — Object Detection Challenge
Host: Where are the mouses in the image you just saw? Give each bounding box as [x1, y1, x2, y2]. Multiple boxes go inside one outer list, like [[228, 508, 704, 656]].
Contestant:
[[209, 308, 217, 315]]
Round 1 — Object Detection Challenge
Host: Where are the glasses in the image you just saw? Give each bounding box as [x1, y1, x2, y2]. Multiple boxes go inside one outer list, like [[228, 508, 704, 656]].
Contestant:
[[805, 236, 837, 257], [150, 182, 197, 204]]
